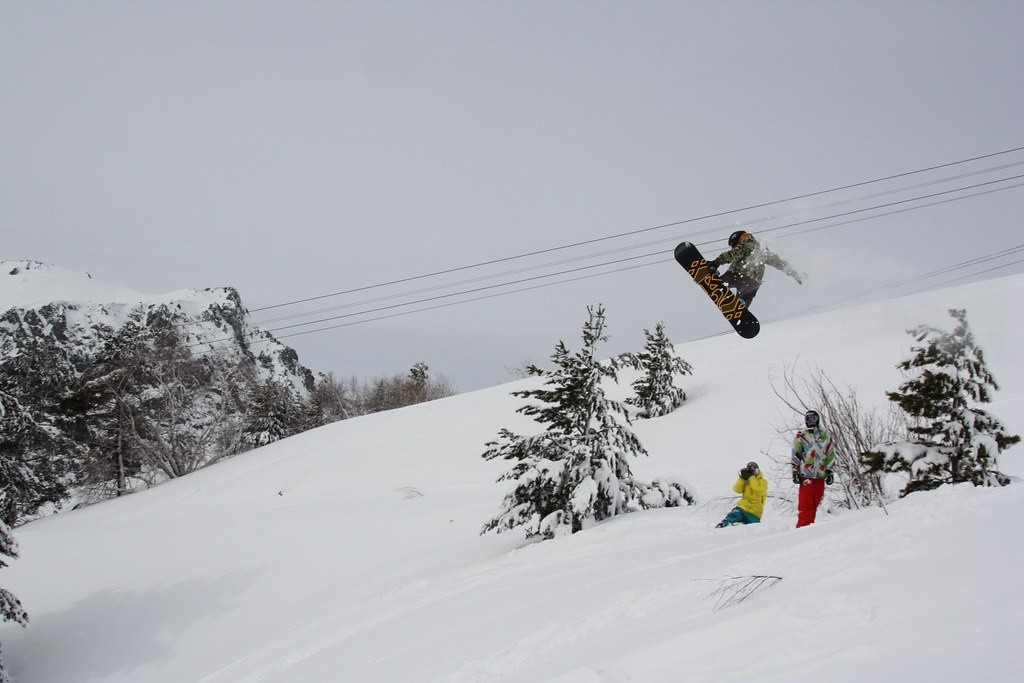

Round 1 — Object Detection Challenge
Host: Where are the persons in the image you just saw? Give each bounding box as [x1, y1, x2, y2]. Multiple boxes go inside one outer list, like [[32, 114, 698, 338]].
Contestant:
[[706, 230, 802, 307], [790, 410, 838, 530], [716, 461, 768, 527]]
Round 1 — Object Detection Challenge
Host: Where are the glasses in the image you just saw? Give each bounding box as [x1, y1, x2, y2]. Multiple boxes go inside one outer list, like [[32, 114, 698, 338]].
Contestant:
[[805, 415, 818, 421], [747, 462, 758, 468]]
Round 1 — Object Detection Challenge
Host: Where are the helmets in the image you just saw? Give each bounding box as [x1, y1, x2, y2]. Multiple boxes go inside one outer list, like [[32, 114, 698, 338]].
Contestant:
[[747, 462, 759, 474], [728, 231, 747, 248], [805, 410, 820, 427]]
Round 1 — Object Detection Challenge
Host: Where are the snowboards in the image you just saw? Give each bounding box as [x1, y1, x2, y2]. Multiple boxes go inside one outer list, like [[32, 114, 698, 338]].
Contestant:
[[673, 240, 760, 339]]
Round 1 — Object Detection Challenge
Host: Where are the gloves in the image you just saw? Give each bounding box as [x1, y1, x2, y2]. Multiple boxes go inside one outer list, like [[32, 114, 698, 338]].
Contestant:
[[825, 472, 834, 485], [707, 260, 720, 271], [792, 474, 800, 484], [789, 269, 802, 285], [741, 469, 751, 479]]
